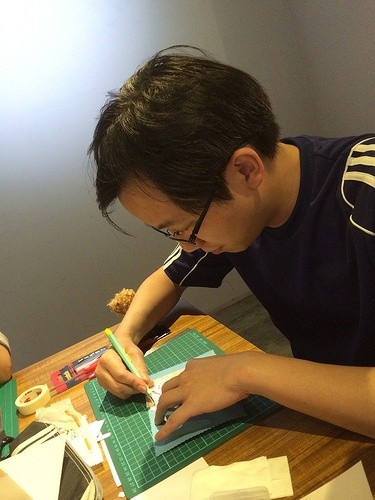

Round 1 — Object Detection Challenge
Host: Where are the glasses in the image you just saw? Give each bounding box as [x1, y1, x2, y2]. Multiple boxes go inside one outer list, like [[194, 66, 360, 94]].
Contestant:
[[144, 203, 211, 247]]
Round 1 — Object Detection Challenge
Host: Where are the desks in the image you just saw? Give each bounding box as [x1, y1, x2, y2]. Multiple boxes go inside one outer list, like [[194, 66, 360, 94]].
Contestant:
[[8, 298, 375, 500]]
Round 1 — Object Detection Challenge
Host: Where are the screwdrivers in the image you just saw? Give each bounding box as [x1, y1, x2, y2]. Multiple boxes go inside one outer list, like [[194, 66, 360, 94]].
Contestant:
[[49, 360, 98, 392]]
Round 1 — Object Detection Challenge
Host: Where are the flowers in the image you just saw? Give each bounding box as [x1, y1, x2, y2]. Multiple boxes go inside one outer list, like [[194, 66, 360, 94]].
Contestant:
[[106, 288, 135, 315]]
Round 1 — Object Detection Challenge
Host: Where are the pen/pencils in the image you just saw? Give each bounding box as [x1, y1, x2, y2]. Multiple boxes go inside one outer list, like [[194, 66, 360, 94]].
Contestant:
[[104, 327, 156, 404]]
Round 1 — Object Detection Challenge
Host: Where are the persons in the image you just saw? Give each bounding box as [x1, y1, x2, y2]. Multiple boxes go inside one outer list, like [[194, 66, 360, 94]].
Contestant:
[[88, 45, 375, 441]]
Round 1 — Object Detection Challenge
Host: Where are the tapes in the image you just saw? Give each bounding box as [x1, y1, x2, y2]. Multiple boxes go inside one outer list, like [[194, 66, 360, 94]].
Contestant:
[[14, 384, 51, 415]]
